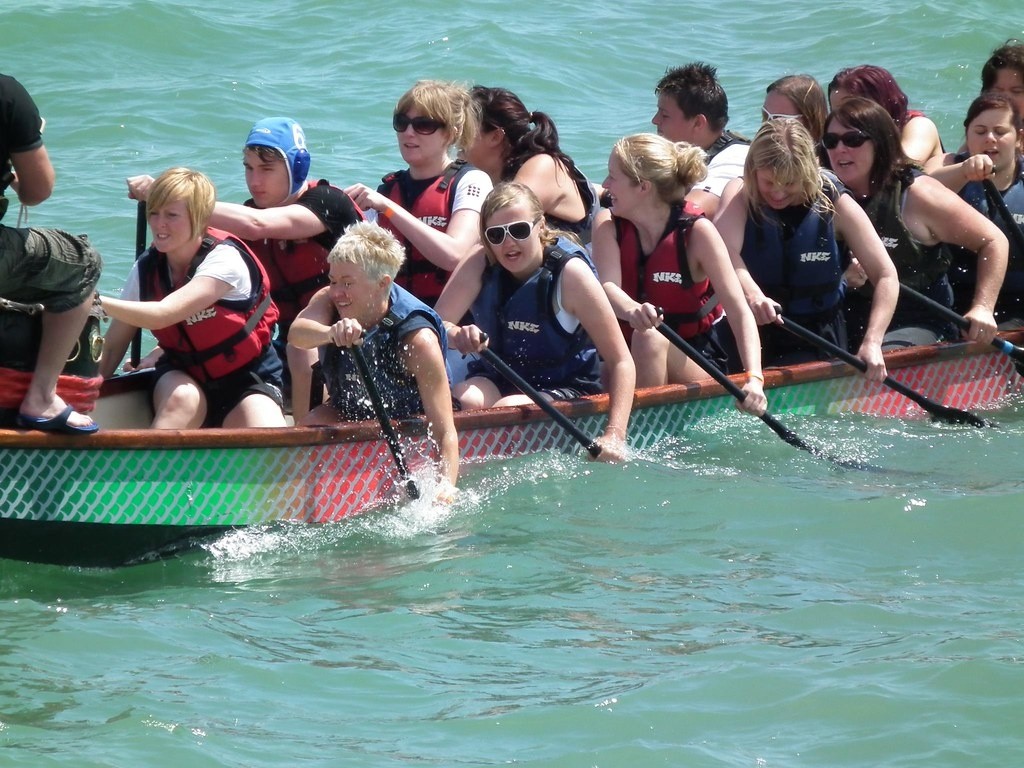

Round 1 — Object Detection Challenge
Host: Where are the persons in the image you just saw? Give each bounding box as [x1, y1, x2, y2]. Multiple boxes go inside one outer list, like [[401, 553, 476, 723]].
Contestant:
[[430, 182, 636, 460], [99, 166, 285, 430], [127, 116, 366, 415], [458, 86, 600, 248], [0, 74, 102, 433], [650, 64, 754, 220], [820, 95, 1010, 354], [590, 133, 770, 416], [288, 218, 459, 501], [343, 80, 493, 326], [828, 64, 944, 164], [958, 38, 1024, 155], [924, 93, 1024, 326], [761, 73, 828, 163], [713, 116, 900, 383]]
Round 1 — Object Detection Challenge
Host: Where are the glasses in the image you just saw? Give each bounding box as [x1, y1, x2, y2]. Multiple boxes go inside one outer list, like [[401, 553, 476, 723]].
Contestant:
[[761, 105, 803, 123], [822, 131, 872, 149], [484, 214, 542, 247], [393, 113, 447, 135]]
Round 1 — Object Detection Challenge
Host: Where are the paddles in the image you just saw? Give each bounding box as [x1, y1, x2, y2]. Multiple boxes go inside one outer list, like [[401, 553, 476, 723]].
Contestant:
[[332, 328, 420, 499], [774, 305, 999, 427], [480, 333, 601, 458], [983, 166, 1024, 251], [655, 306, 790, 440], [900, 281, 1024, 377], [131, 201, 147, 369]]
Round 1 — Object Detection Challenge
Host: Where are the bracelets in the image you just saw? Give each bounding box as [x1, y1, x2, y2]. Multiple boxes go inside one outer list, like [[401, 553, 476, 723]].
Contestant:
[[385, 202, 399, 217], [744, 370, 764, 382], [445, 322, 455, 334]]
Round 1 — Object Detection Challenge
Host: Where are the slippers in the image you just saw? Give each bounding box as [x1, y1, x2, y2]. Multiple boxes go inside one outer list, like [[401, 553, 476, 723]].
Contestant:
[[15, 403, 99, 435]]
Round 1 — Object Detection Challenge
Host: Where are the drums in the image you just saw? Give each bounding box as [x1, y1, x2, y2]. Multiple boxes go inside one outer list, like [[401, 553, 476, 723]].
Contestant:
[[0, 290, 109, 429]]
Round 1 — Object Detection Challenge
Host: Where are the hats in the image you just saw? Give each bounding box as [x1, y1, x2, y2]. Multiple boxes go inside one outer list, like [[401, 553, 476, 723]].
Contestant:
[[245, 117, 311, 196]]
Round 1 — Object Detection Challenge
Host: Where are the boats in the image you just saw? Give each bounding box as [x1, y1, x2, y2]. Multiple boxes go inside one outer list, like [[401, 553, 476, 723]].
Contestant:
[[0, 316, 1024, 550]]
